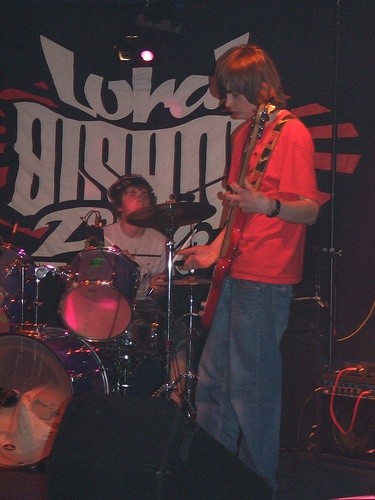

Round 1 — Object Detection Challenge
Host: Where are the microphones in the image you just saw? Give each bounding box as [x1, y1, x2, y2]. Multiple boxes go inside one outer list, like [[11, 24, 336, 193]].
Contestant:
[[94, 216, 104, 247], [0, 389, 21, 408], [169, 192, 195, 202]]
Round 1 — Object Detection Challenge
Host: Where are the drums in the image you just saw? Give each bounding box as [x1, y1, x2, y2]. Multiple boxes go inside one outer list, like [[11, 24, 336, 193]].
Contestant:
[[0, 241, 45, 334], [112, 307, 170, 357], [0, 325, 110, 469], [58, 246, 141, 343]]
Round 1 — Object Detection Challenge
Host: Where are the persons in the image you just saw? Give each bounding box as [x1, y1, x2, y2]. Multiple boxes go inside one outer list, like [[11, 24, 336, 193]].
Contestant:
[[172, 44, 318, 500], [82, 173, 170, 298]]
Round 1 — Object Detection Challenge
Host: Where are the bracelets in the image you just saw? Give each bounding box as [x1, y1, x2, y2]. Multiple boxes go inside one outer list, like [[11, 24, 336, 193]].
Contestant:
[[267, 199, 282, 218]]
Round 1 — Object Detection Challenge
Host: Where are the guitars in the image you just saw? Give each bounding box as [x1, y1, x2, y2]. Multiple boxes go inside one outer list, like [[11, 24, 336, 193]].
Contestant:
[[199, 100, 275, 328]]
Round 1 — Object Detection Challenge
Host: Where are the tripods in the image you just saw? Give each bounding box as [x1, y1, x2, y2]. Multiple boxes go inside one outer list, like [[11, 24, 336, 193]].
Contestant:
[[151, 211, 199, 427]]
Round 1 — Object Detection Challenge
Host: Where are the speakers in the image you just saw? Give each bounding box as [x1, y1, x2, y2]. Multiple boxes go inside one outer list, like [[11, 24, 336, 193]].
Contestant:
[[315, 366, 375, 471]]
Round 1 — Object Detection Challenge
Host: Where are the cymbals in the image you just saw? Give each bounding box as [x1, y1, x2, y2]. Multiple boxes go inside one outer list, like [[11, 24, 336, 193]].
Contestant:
[[151, 276, 211, 286], [124, 201, 217, 229]]
